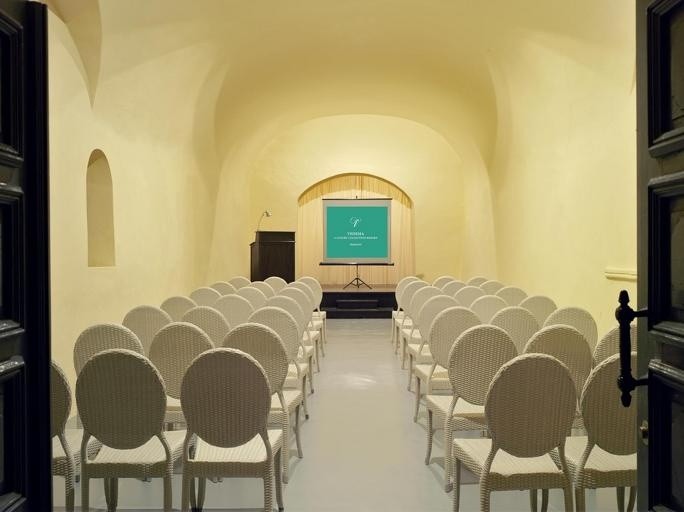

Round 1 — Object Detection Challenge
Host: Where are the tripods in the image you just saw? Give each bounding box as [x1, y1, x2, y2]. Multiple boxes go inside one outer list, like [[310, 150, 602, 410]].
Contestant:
[[343, 265, 372, 289]]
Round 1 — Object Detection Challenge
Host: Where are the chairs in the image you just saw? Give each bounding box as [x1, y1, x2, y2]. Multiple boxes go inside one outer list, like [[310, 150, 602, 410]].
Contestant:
[[388, 274, 640, 511], [48, 276, 328, 512]]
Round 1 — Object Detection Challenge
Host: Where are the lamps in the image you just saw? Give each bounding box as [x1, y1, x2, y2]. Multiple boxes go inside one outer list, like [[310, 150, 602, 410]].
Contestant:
[[255, 209, 272, 231]]
